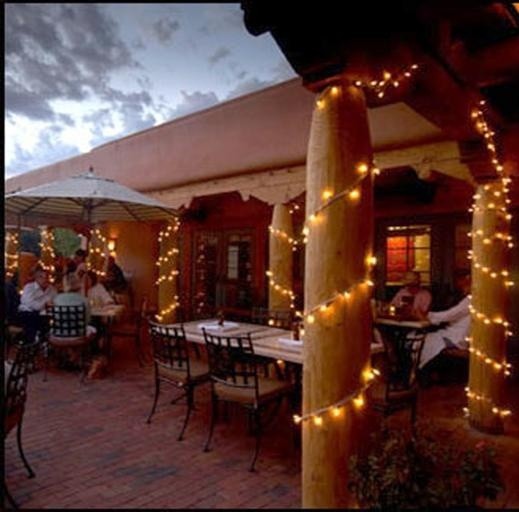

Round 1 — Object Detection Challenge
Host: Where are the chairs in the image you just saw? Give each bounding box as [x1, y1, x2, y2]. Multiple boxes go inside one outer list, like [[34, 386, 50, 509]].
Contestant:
[[4, 292, 145, 385], [3, 342, 42, 508], [146, 307, 427, 474]]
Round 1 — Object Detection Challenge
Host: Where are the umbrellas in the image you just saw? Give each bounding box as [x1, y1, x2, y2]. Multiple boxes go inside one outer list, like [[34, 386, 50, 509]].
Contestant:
[[3, 167, 183, 250]]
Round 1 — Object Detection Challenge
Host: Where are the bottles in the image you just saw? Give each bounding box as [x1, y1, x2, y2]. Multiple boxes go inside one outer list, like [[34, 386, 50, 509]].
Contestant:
[[291, 316, 301, 341], [216, 308, 224, 327]]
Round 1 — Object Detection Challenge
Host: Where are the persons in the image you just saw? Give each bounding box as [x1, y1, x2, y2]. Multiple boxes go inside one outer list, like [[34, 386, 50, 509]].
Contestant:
[[3, 249, 128, 380], [371, 271, 472, 388]]
